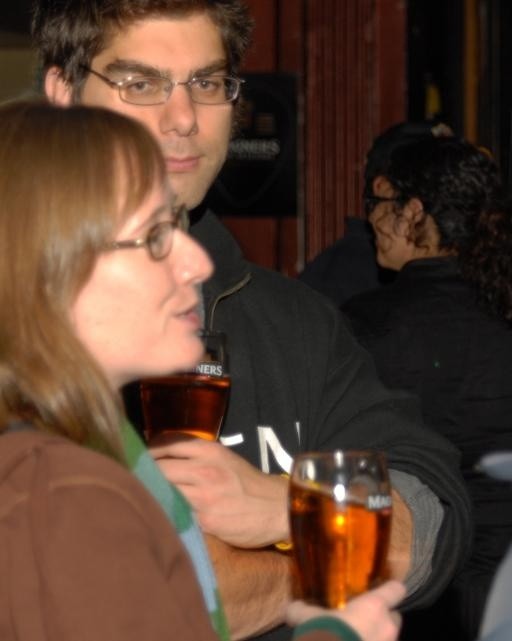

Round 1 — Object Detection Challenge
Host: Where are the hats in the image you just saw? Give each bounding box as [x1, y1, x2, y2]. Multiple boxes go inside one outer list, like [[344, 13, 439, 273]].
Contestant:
[[364, 116, 494, 181]]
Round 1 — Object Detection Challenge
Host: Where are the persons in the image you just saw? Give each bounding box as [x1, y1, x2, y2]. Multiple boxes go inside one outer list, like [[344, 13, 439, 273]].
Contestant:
[[0, 99, 406, 641], [27, 0, 471, 641], [296, 119, 456, 326], [366, 136, 512, 640]]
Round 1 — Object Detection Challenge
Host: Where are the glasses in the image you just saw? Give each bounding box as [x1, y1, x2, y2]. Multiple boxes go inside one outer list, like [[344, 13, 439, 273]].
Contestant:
[[75, 62, 245, 105], [99, 202, 190, 260], [363, 190, 399, 211]]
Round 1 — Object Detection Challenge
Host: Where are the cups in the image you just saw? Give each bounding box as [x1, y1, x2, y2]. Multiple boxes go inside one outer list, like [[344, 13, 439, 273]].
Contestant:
[[141, 328, 230, 450], [288, 453, 392, 609]]
